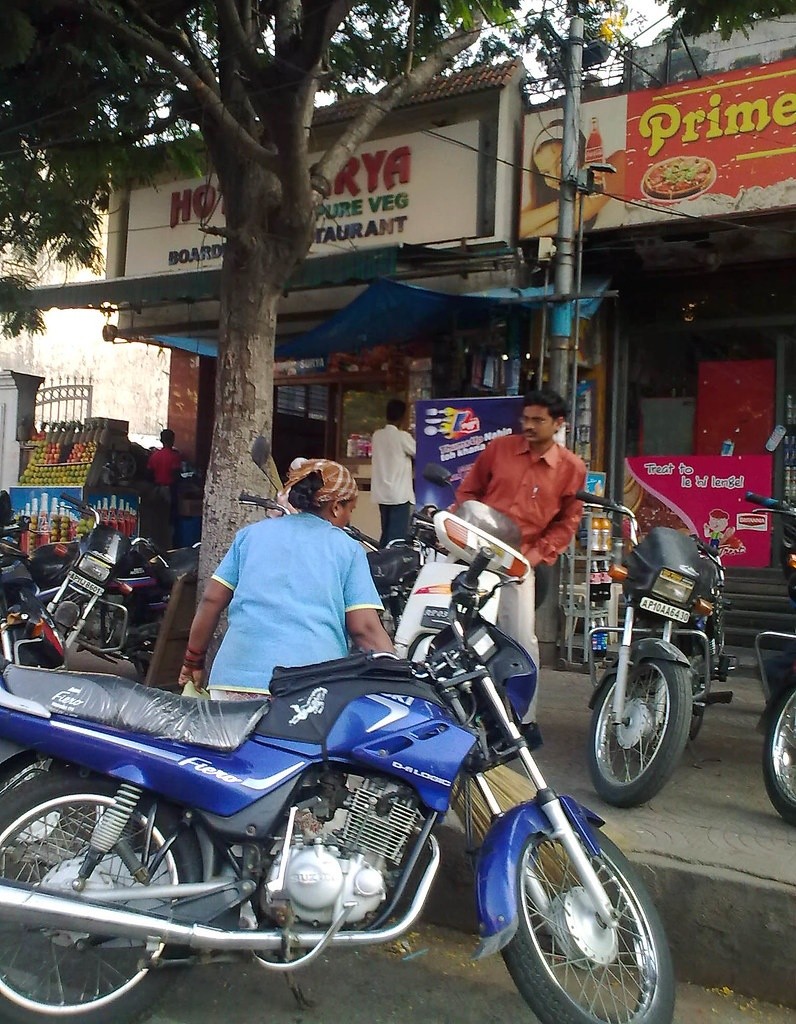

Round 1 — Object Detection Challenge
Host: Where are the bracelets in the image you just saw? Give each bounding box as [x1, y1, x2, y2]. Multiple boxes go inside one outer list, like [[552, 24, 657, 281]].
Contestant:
[[183, 646, 207, 670]]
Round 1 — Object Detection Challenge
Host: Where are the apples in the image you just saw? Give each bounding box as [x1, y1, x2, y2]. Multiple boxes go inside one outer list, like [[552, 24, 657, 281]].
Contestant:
[[15, 430, 97, 487], [27, 516, 97, 554]]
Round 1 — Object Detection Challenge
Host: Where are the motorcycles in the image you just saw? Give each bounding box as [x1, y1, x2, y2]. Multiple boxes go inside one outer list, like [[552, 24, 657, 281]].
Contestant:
[[569, 489, 744, 810], [0, 546, 679, 1024], [742, 487, 795, 828], [0, 485, 209, 682], [244, 430, 551, 653]]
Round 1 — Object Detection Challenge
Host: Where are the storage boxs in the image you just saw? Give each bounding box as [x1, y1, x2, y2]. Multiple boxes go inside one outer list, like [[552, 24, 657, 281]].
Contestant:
[[35, 442, 102, 487]]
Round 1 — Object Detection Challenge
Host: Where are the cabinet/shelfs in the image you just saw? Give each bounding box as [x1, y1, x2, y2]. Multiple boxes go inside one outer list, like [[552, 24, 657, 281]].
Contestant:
[[561, 504, 619, 673]]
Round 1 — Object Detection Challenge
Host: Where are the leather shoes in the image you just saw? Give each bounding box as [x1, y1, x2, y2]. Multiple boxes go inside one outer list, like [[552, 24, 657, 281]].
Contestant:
[[521, 723, 543, 745]]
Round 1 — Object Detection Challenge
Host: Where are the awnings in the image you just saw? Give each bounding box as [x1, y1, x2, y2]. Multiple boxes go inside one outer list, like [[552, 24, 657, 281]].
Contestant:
[[28, 238, 491, 313]]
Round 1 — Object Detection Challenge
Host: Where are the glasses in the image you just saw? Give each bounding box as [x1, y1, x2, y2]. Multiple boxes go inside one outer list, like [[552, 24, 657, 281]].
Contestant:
[[515, 416, 555, 426]]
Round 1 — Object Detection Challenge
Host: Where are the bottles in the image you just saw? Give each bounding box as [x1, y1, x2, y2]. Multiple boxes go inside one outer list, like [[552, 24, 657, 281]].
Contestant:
[[10, 492, 139, 564], [557, 506, 638, 663], [345, 434, 372, 458]]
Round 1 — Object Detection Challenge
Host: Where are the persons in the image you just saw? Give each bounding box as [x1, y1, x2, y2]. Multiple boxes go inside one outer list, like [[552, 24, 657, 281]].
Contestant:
[[449, 388, 587, 748], [178, 455, 395, 701], [146, 428, 186, 548], [370, 398, 415, 547], [519, 119, 627, 238]]
